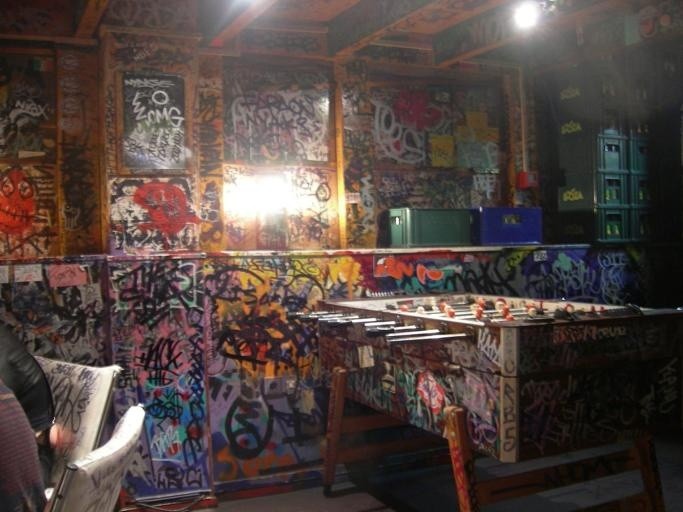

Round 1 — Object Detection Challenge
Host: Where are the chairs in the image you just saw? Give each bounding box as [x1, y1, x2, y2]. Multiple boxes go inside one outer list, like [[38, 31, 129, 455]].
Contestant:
[[40, 404, 146, 512], [32, 354, 124, 489]]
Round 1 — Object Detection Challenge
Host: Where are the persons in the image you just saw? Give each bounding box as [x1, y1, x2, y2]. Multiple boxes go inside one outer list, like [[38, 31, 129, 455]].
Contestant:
[[1, 315, 56, 488], [386, 294, 605, 325], [0, 375, 66, 512]]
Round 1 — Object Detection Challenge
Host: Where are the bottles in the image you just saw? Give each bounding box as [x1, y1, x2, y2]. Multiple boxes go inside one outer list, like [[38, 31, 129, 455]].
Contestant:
[[605, 52, 651, 239]]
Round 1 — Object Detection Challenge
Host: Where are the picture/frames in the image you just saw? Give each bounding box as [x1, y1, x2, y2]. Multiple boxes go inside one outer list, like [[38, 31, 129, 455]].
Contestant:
[[111, 66, 196, 178]]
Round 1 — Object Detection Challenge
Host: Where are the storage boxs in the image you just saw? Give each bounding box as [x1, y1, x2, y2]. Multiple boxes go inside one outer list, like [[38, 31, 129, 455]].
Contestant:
[[384, 206, 472, 249], [552, 71, 659, 246], [468, 204, 543, 247]]
